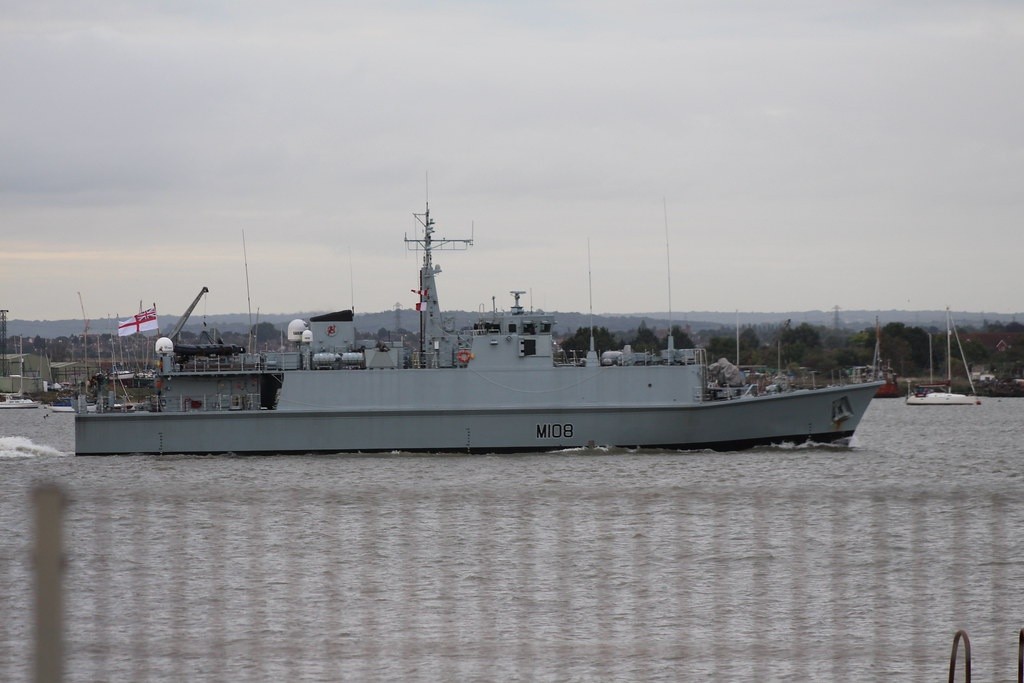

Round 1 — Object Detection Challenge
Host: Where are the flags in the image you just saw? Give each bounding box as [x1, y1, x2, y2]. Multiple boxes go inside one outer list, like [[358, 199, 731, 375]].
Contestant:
[[411, 288, 428, 297], [118, 307, 158, 337]]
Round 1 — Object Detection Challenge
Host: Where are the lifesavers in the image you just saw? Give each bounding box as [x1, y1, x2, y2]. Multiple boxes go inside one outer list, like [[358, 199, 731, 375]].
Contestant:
[[458, 351, 470, 363], [161, 400, 166, 406]]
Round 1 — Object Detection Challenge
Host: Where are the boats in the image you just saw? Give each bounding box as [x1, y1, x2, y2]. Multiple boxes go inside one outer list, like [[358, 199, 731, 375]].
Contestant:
[[71, 174, 889, 455]]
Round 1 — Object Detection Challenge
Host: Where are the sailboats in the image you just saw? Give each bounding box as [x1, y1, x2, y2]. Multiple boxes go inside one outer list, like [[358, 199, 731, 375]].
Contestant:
[[907, 306, 979, 405], [849, 317, 900, 398]]
